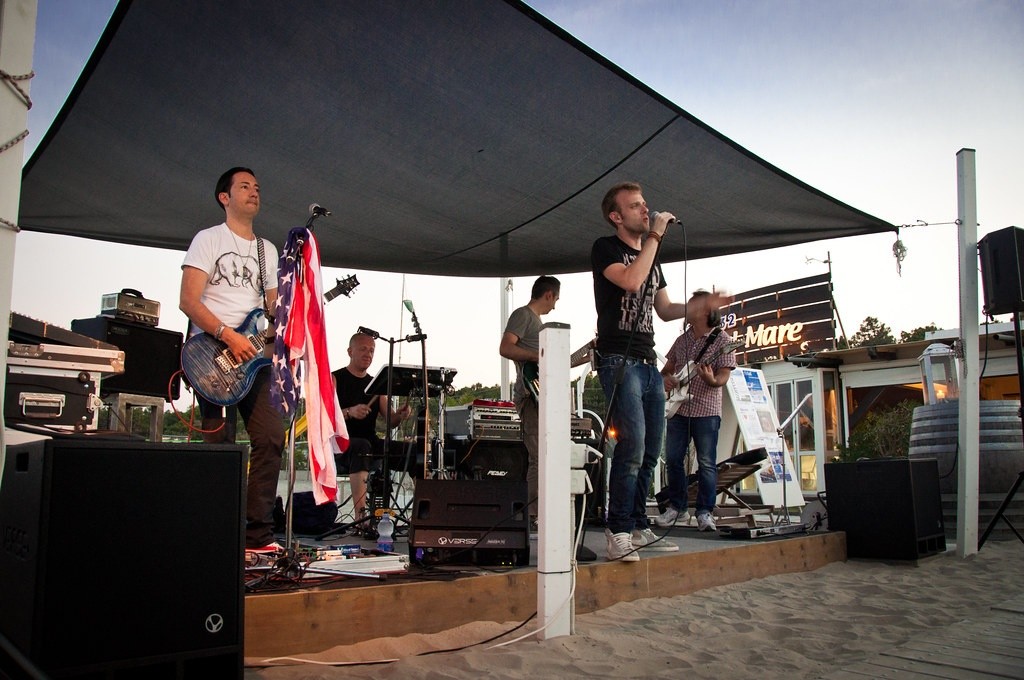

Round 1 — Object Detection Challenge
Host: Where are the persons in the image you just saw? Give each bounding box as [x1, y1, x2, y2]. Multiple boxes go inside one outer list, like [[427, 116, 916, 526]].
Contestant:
[[500, 276, 590, 530], [179, 166, 287, 560], [331, 332, 418, 539], [654, 291, 736, 534], [589, 183, 735, 561]]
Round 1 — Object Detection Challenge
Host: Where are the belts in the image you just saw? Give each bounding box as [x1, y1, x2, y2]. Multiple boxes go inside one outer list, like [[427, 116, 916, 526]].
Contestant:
[[629, 354, 657, 365]]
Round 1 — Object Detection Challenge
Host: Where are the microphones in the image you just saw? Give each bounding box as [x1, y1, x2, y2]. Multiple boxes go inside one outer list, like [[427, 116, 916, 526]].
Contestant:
[[395, 334, 427, 343], [650, 211, 683, 225], [359, 326, 389, 342], [312, 204, 323, 213]]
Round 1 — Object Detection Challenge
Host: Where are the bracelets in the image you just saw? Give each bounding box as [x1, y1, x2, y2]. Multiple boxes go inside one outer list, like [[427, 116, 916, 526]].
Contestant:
[[214, 323, 226, 341], [647, 231, 661, 242], [347, 407, 352, 419]]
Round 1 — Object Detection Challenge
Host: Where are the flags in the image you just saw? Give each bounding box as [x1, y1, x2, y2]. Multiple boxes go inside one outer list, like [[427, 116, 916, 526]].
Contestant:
[[267, 226, 350, 505]]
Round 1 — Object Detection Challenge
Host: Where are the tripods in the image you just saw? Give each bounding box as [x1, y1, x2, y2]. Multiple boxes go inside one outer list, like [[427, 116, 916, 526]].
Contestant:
[[313, 337, 409, 541]]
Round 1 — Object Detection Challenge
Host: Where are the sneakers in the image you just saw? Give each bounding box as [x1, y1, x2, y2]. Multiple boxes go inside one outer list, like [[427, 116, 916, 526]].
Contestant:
[[630, 528, 679, 551], [697, 512, 716, 531], [654, 506, 690, 526], [604, 528, 640, 561], [246, 540, 287, 562]]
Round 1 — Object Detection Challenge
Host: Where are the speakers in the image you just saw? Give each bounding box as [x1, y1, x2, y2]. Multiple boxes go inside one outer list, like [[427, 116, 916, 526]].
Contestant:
[[823, 457, 946, 561], [407, 440, 531, 565], [71, 316, 184, 401], [978, 226, 1024, 315], [275, 490, 339, 536], [0, 436, 249, 680]]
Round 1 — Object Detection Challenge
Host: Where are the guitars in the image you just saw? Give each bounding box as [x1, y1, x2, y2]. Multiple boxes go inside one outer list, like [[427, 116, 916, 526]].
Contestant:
[[403, 299, 431, 478], [662, 338, 746, 419], [522, 333, 599, 406], [181, 273, 361, 407]]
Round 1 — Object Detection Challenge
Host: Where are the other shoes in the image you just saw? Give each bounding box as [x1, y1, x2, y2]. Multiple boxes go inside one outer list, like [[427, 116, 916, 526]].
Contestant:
[[530, 520, 538, 533]]
